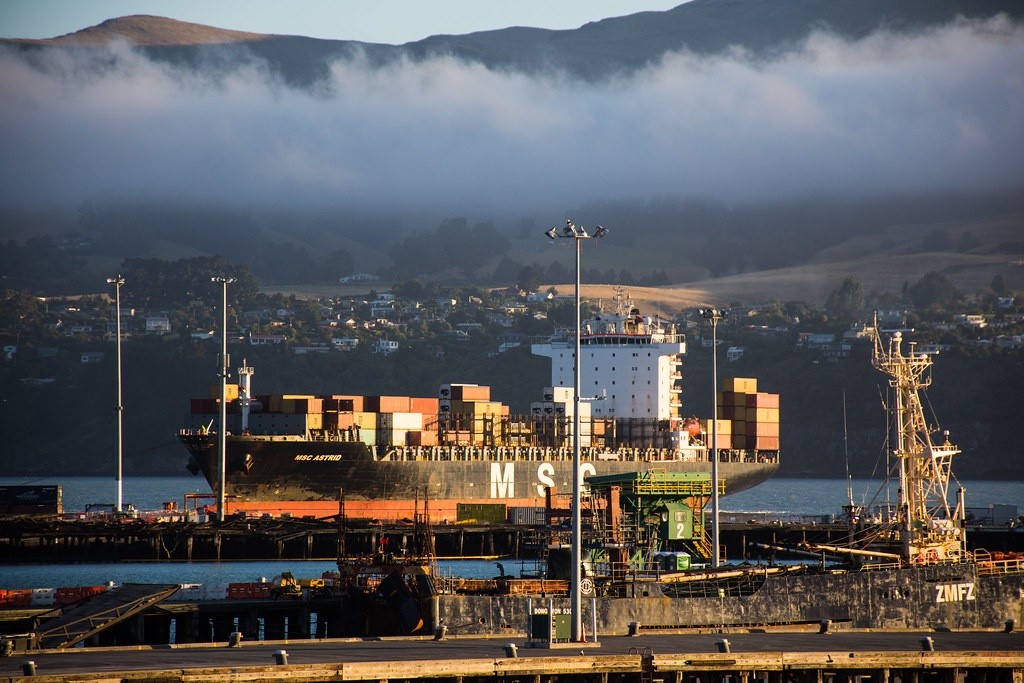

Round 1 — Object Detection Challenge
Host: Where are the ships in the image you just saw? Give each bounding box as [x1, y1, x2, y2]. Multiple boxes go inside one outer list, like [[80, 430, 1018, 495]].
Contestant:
[[173, 287, 784, 512]]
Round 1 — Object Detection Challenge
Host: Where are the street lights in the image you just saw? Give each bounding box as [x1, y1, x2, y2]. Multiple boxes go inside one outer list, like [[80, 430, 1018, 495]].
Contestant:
[[107, 274, 127, 512], [541, 214, 611, 643], [211, 274, 237, 520], [697, 307, 726, 569]]
